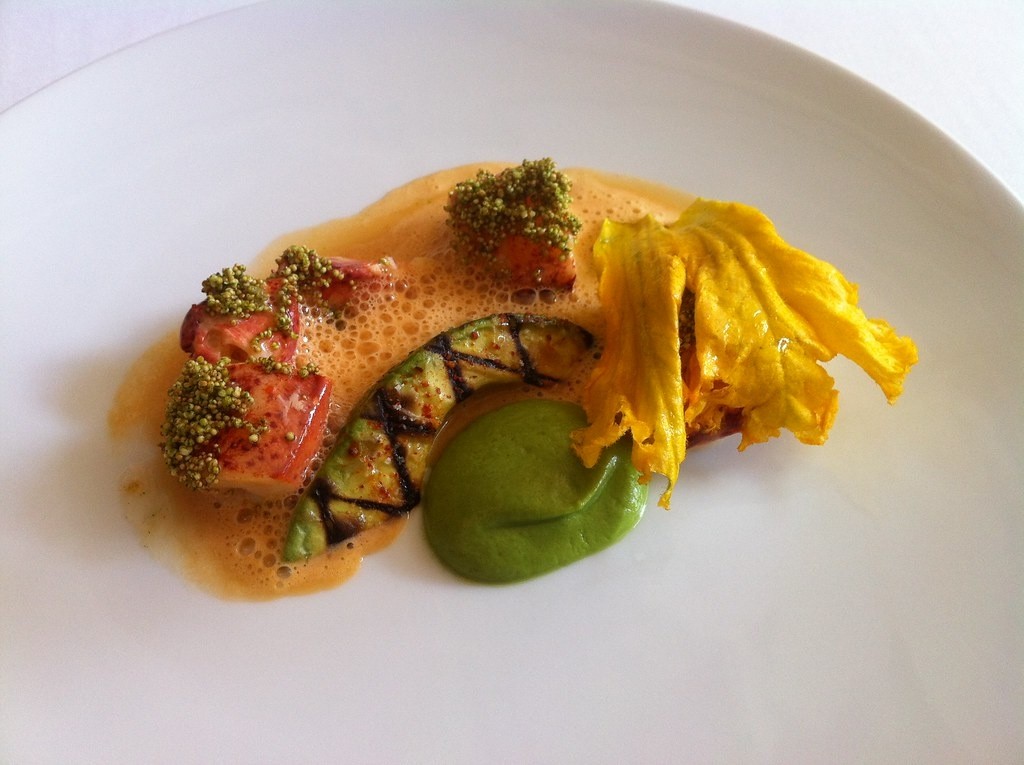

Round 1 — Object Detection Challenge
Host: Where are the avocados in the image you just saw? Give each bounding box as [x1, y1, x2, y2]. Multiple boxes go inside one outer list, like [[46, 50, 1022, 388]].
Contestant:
[[282, 314, 597, 564]]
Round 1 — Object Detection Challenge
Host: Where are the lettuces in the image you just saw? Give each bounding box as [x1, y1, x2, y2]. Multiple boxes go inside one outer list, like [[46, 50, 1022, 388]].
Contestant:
[[569, 197, 918, 508]]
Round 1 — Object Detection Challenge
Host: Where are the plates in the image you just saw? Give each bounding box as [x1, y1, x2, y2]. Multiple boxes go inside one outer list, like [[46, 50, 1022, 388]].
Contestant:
[[0, 2, 1022, 762]]
